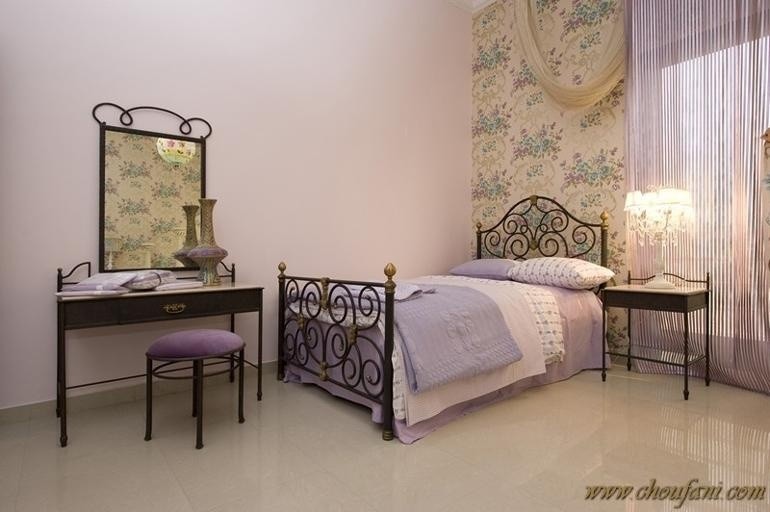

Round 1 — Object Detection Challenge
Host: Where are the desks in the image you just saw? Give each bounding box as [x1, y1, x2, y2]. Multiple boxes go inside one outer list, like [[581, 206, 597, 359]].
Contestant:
[[601, 271, 712, 403], [55, 257, 265, 450]]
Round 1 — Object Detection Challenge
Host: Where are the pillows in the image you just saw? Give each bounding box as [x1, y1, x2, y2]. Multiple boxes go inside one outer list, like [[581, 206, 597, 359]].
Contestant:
[[447, 258, 521, 280], [508, 257, 614, 290]]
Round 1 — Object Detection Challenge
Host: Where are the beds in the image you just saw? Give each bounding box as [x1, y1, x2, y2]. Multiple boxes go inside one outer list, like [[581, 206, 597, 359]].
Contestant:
[[275, 191, 608, 445]]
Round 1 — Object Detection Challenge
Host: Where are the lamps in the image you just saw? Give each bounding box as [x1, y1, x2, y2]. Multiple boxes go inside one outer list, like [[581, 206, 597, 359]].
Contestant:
[[625, 182, 696, 288], [154, 138, 197, 170]]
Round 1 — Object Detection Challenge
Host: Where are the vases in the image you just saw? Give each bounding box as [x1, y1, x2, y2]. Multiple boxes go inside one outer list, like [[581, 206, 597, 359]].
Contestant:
[[186, 198, 229, 285], [173, 204, 199, 267]]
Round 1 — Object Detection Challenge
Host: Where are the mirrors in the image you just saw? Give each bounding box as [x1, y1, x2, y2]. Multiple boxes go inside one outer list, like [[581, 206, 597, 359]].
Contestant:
[[91, 101, 213, 273]]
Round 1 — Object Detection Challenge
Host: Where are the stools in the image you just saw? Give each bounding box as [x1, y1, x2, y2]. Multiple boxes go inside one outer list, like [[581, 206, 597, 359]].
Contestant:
[[143, 329, 247, 450]]
